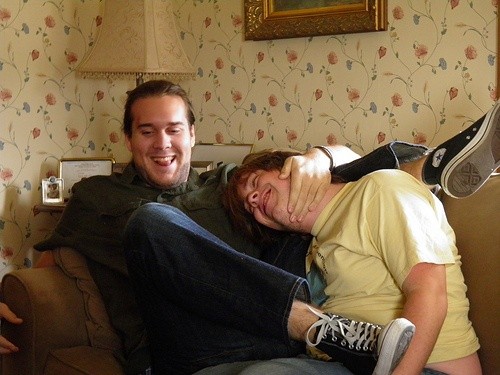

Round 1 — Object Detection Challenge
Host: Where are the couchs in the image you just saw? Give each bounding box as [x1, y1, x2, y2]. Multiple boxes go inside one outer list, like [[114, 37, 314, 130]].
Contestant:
[[0, 174, 500, 375]]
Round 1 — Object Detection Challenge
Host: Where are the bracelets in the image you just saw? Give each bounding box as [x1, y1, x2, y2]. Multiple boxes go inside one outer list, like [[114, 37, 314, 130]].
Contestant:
[[314, 145, 334, 172]]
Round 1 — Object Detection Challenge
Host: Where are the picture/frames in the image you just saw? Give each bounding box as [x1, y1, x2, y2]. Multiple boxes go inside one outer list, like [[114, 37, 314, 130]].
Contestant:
[[42, 144, 257, 205], [243, 0, 389, 41]]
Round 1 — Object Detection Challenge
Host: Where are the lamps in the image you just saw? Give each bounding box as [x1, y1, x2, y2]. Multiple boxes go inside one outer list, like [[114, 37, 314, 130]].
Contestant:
[[74, 1, 195, 88]]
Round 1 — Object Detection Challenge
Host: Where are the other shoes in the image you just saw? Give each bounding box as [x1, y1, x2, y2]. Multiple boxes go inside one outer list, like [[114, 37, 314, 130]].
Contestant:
[[421, 97, 500, 200], [305, 304, 415, 375]]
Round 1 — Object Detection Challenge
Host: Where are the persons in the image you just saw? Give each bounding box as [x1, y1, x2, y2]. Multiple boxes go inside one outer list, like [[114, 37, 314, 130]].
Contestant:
[[190, 147, 483, 375], [1, 80, 500, 375]]
